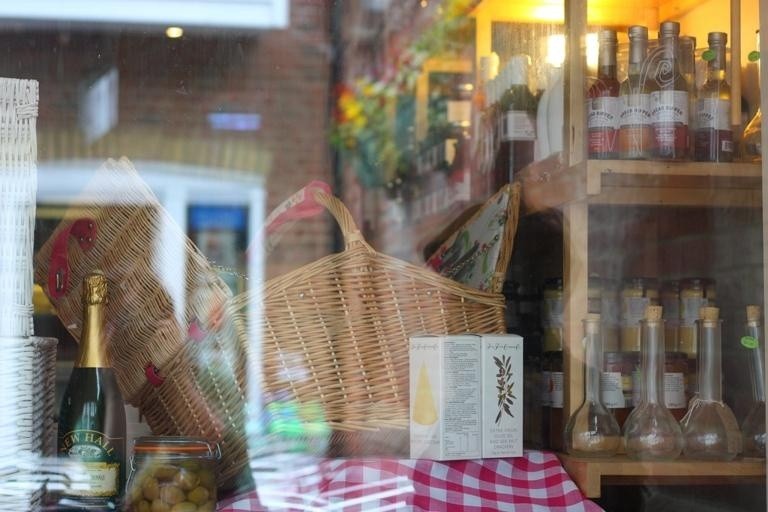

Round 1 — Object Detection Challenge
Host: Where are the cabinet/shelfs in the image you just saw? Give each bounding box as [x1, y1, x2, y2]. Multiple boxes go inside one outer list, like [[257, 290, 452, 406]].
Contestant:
[[367, 0, 768, 508]]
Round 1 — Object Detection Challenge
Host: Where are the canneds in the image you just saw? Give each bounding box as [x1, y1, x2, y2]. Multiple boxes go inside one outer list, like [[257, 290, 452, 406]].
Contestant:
[[124, 436, 223, 512], [503, 275, 717, 448]]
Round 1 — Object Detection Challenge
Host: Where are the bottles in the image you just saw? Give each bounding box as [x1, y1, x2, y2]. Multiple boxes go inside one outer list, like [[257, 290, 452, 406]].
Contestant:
[[56, 271, 222, 510], [468, 25, 732, 201], [564, 308, 767, 460]]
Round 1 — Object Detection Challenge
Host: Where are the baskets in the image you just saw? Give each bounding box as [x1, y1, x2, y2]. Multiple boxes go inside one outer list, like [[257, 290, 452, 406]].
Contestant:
[[35, 156, 509, 493]]
